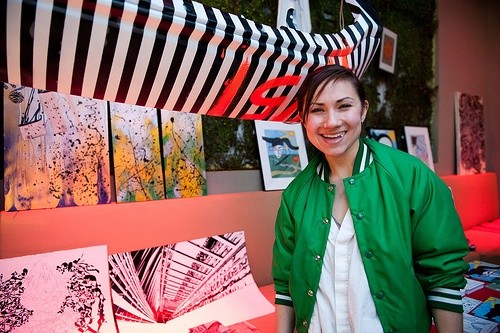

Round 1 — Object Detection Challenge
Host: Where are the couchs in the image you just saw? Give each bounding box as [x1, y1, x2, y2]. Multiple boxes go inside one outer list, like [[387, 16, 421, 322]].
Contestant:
[[439, 172, 500, 263]]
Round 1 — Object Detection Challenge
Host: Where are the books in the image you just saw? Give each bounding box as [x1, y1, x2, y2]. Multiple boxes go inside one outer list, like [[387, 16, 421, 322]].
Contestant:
[[464, 277, 485, 294]]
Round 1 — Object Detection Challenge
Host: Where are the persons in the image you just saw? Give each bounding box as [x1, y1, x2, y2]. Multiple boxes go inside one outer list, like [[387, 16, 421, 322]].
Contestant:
[[272, 65, 470, 333]]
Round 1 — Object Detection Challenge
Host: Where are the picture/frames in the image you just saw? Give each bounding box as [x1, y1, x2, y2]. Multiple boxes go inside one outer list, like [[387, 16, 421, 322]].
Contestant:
[[252, 119, 312, 192], [369, 124, 436, 174], [376, 25, 399, 77]]
